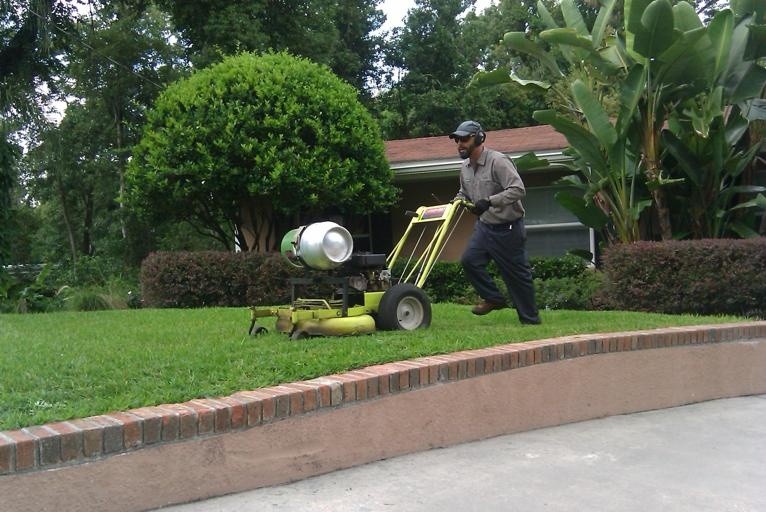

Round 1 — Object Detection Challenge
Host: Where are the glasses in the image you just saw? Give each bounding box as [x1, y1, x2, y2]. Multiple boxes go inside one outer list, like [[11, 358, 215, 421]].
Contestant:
[[454, 135, 470, 143]]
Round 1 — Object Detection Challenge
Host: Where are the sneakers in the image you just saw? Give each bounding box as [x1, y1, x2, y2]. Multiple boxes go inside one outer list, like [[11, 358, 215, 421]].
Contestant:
[[472, 300, 505, 315]]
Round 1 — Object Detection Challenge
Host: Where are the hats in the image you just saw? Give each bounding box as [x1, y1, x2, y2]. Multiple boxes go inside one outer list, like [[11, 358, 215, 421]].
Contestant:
[[449, 121, 486, 144]]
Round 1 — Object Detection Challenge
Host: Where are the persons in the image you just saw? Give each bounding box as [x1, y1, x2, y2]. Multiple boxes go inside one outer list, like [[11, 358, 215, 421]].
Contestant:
[[449, 119, 541, 325]]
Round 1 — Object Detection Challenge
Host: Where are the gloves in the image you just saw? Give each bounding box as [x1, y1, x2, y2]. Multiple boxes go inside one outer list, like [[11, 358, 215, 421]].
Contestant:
[[469, 199, 489, 215]]
